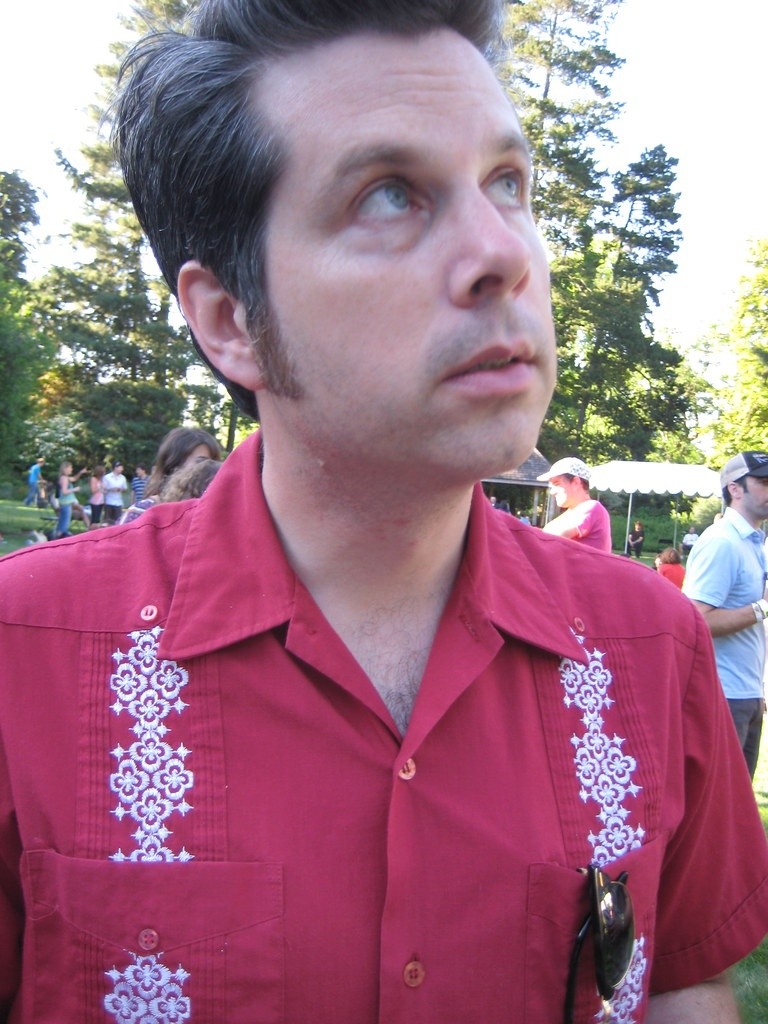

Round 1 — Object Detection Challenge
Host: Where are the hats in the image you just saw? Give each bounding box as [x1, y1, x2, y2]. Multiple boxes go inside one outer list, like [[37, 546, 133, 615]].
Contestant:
[[536, 457, 591, 482], [720, 450, 768, 490]]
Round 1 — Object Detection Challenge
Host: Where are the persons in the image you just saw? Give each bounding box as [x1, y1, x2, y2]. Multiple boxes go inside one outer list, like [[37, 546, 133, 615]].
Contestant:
[[21, 427, 227, 543], [680, 451, 768, 784], [621, 520, 644, 558], [681, 526, 699, 561], [543, 457, 612, 553], [0, 0, 768, 1024], [652, 547, 686, 588], [490, 495, 532, 528]]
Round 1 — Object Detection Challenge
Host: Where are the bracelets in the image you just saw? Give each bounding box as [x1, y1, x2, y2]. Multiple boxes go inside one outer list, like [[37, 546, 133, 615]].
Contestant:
[[751, 598, 768, 623]]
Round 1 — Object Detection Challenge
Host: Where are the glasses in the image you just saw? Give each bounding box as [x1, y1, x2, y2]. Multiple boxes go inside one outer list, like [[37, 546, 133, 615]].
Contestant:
[[564, 864, 634, 1024]]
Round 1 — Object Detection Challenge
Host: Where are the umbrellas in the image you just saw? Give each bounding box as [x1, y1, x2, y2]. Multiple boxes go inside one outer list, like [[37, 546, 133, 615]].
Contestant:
[[581, 459, 724, 556]]
